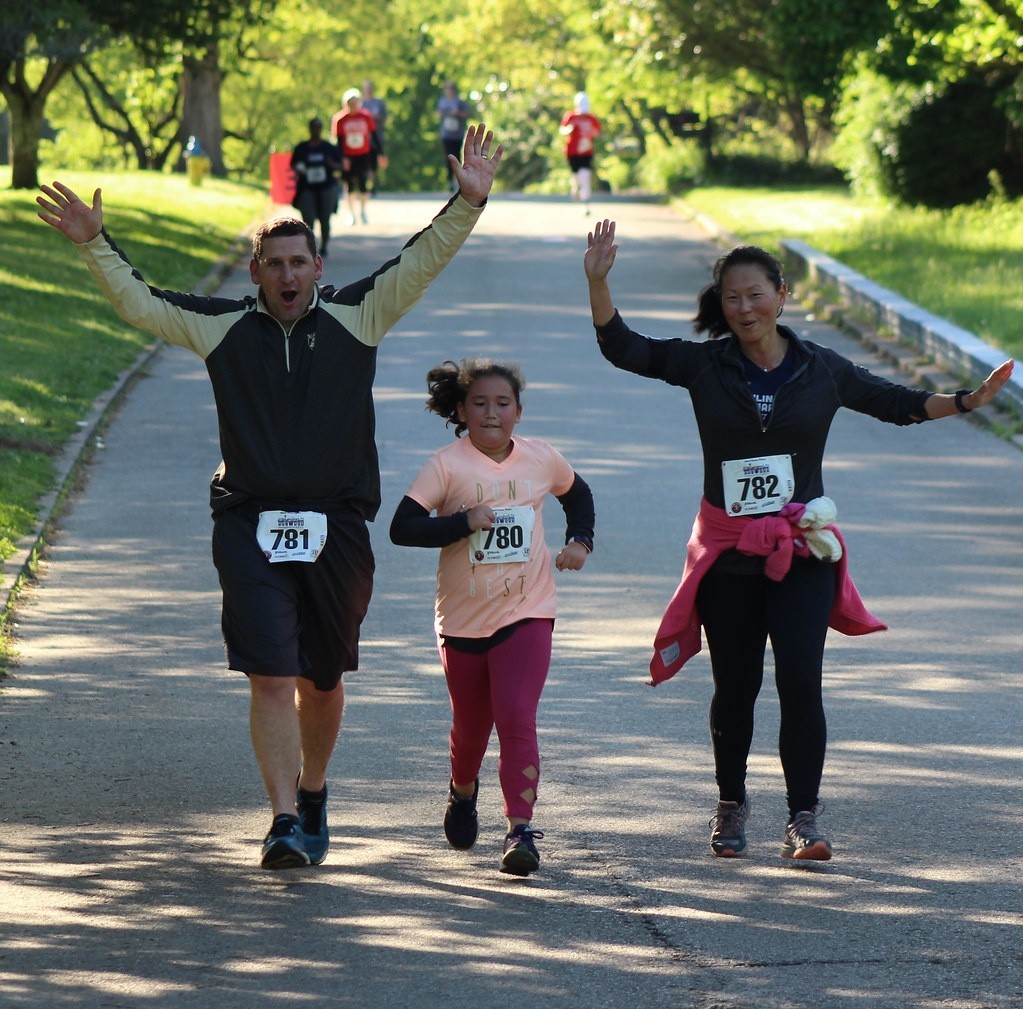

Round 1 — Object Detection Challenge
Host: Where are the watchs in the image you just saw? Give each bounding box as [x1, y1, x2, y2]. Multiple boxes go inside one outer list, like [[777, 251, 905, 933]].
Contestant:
[[955, 389, 975, 413]]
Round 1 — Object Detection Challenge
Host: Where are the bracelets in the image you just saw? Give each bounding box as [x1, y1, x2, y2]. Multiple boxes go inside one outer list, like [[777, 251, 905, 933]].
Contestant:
[[567, 534, 594, 552]]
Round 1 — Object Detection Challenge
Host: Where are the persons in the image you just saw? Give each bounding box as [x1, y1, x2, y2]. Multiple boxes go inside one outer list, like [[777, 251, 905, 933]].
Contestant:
[[290, 119, 344, 257], [389, 360, 596, 875], [559, 92, 601, 200], [360, 81, 386, 193], [36, 122, 504, 867], [334, 95, 384, 225], [437, 81, 468, 190], [583, 216, 1015, 859]]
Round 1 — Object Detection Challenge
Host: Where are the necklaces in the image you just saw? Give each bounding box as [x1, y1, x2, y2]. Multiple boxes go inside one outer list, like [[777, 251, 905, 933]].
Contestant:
[[751, 356, 778, 371]]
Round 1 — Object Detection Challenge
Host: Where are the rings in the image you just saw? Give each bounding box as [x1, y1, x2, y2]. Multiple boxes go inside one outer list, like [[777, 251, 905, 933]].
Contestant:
[[482, 156, 488, 159]]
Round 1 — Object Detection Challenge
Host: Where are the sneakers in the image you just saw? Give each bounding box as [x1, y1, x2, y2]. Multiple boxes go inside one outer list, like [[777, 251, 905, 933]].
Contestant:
[[498, 824, 544, 876], [296, 770, 331, 864], [708, 793, 750, 857], [443, 776, 480, 850], [780, 804, 832, 860], [259, 814, 309, 867]]
